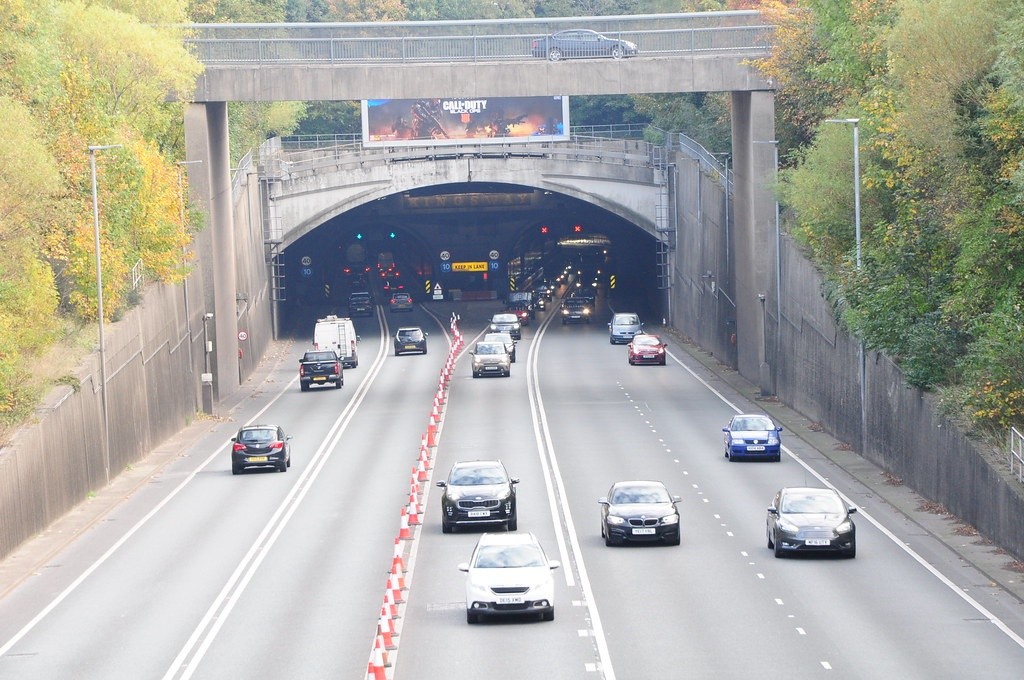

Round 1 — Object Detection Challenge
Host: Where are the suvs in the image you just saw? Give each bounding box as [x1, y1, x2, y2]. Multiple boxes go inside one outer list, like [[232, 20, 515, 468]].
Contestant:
[[314, 314, 362, 369], [298, 349, 346, 391], [346, 292, 375, 316], [435, 459, 520, 534], [607, 313, 645, 344]]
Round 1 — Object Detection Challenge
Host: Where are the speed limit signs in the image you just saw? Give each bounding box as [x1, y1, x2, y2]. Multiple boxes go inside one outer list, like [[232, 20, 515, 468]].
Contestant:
[[302, 256, 312, 267], [488, 261, 500, 270], [440, 251, 451, 261], [441, 262, 451, 272], [239, 331, 248, 342], [489, 250, 500, 261]]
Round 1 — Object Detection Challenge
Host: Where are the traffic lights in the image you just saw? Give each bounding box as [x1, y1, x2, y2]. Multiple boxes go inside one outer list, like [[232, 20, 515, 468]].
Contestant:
[[390, 232, 397, 239], [355, 233, 363, 241], [541, 226, 548, 234], [574, 225, 583, 232]]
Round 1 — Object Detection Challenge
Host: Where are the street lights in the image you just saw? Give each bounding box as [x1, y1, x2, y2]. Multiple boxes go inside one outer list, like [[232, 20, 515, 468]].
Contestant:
[[85, 142, 126, 473], [680, 157, 706, 279], [752, 138, 781, 400], [668, 161, 674, 326], [824, 116, 871, 459], [707, 151, 729, 291], [177, 159, 203, 374]]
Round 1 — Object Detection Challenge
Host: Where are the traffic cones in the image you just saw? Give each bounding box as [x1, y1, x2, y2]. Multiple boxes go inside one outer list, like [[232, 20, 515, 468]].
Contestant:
[[362, 307, 467, 680]]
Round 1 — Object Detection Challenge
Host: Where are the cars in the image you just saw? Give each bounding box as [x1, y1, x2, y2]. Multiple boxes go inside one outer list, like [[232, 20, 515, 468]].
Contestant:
[[389, 292, 414, 312], [531, 29, 638, 61], [391, 327, 428, 355], [483, 333, 518, 364], [341, 260, 405, 296], [230, 425, 294, 475], [768, 487, 858, 557], [456, 530, 561, 625], [598, 481, 683, 547], [626, 333, 669, 367], [468, 341, 512, 377], [489, 313, 523, 340], [723, 413, 784, 462], [499, 256, 602, 325]]
